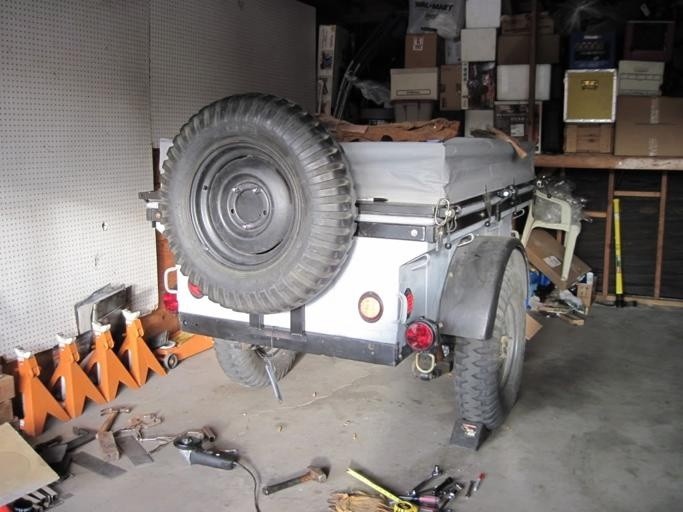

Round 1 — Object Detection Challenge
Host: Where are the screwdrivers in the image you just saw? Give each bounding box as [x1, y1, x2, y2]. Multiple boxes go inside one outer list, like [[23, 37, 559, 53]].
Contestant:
[[398, 496, 441, 512]]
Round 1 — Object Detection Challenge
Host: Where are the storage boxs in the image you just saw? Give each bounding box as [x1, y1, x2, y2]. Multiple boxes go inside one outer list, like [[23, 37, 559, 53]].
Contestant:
[[388, 0, 683, 157]]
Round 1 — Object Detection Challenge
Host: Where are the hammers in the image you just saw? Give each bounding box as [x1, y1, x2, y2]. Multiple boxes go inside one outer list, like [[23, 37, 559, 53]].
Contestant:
[[262, 465, 327, 495], [66, 408, 131, 452]]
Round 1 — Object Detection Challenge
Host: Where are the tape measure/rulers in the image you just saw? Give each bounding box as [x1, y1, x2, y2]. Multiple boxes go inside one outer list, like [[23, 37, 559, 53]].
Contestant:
[[344, 468, 418, 512]]
